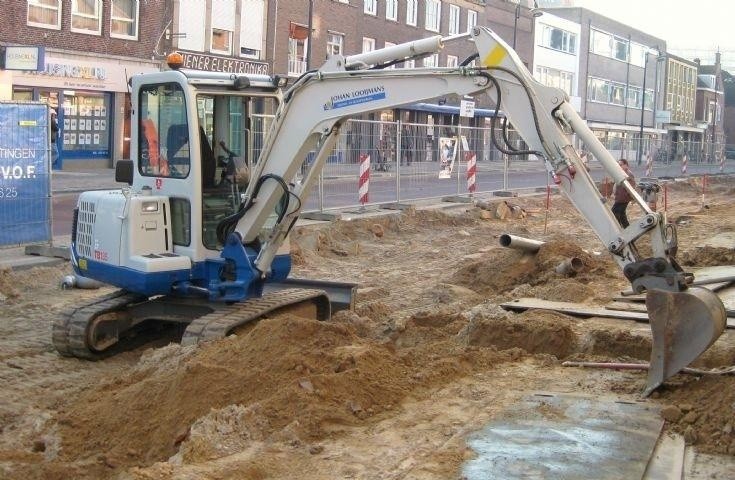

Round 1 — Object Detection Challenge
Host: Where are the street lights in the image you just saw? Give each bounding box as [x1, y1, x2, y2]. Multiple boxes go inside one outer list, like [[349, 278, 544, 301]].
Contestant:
[[638, 44, 667, 165], [513, 0, 543, 51]]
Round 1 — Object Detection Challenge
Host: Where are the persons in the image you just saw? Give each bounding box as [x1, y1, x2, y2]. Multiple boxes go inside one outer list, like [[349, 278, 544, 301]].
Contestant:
[[395, 123, 414, 166], [700, 150, 706, 162], [50, 106, 60, 164], [608, 159, 634, 230]]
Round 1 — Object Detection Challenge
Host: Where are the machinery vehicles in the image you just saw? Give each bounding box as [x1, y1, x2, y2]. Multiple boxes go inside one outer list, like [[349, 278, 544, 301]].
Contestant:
[[51, 26, 726, 400]]
